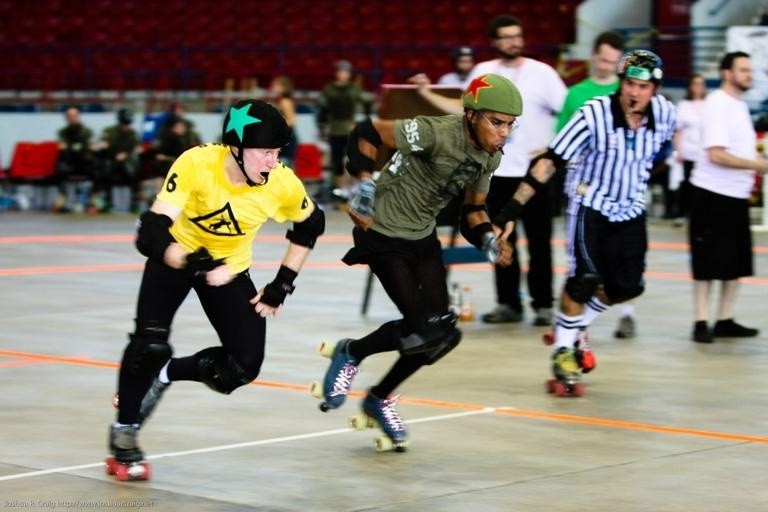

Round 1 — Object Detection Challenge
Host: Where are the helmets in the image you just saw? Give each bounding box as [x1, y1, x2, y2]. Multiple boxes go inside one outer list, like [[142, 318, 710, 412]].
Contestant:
[[616, 49, 665, 85], [463, 74, 522, 116], [223, 99, 290, 149]]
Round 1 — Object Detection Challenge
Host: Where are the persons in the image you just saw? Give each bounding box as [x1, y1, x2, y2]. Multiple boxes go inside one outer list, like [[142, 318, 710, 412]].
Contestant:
[[57, 58, 380, 212], [441, 48, 471, 84], [491, 51, 681, 388], [324, 74, 518, 444], [554, 30, 651, 337], [108, 98, 327, 465], [460, 19, 573, 328], [651, 75, 765, 212], [690, 48, 767, 345]]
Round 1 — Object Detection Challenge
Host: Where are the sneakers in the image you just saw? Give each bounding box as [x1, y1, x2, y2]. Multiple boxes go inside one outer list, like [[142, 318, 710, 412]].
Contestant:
[[694, 318, 756, 342], [615, 315, 635, 337], [484, 303, 522, 323]]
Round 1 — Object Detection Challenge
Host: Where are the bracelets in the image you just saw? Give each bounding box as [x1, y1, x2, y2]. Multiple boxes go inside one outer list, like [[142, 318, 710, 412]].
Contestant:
[[469, 221, 493, 244], [273, 269, 295, 297]]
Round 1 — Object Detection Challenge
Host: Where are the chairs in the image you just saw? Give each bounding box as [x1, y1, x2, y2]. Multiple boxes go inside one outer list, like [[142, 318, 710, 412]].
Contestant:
[[1, 1, 576, 113], [0, 140, 323, 216]]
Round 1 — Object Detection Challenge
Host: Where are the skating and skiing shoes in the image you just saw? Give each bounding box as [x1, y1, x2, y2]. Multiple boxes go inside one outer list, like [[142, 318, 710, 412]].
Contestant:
[[106, 356, 171, 481], [352, 386, 409, 452], [534, 308, 596, 396], [308, 338, 360, 412]]
